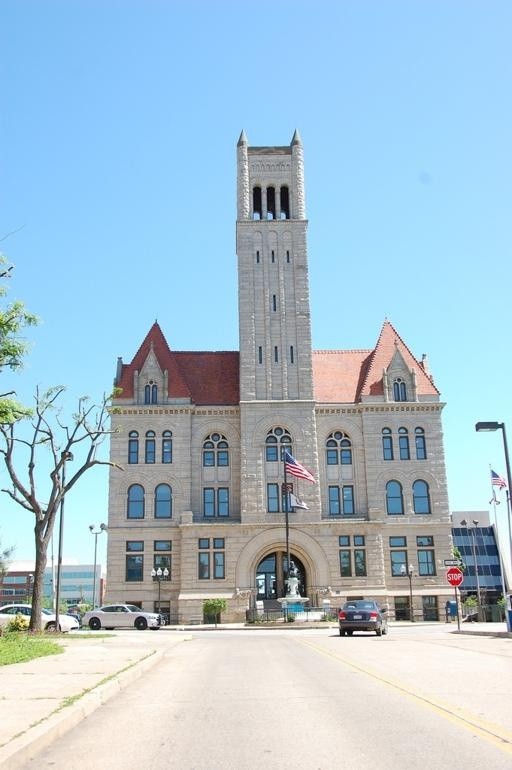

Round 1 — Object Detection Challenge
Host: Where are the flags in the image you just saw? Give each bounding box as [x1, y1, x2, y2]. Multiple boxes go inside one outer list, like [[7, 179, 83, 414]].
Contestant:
[[289, 492, 311, 509], [491, 470, 507, 489], [285, 452, 316, 484]]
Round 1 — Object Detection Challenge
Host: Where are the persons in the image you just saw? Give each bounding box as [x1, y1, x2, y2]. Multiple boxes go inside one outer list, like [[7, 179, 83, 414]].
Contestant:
[[288, 560, 299, 576]]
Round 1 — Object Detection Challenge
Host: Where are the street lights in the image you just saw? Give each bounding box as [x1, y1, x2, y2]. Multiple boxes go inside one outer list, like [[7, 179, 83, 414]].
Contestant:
[[89, 523, 107, 610], [400, 563, 414, 620], [460, 519, 483, 622], [475, 421, 511, 514], [151, 567, 169, 613], [55, 451, 74, 631]]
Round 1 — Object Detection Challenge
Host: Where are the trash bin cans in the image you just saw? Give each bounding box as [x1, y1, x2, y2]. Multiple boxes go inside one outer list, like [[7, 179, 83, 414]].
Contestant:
[[505, 594, 512, 633], [485, 604, 502, 622]]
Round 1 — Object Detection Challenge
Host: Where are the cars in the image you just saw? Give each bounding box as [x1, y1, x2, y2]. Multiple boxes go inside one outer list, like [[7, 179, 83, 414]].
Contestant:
[[338, 600, 388, 636], [81, 604, 162, 630], [0, 604, 79, 634]]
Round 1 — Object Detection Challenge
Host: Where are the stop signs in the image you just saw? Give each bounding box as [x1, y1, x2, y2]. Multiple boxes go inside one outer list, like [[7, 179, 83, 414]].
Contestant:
[[446, 568, 463, 586]]
